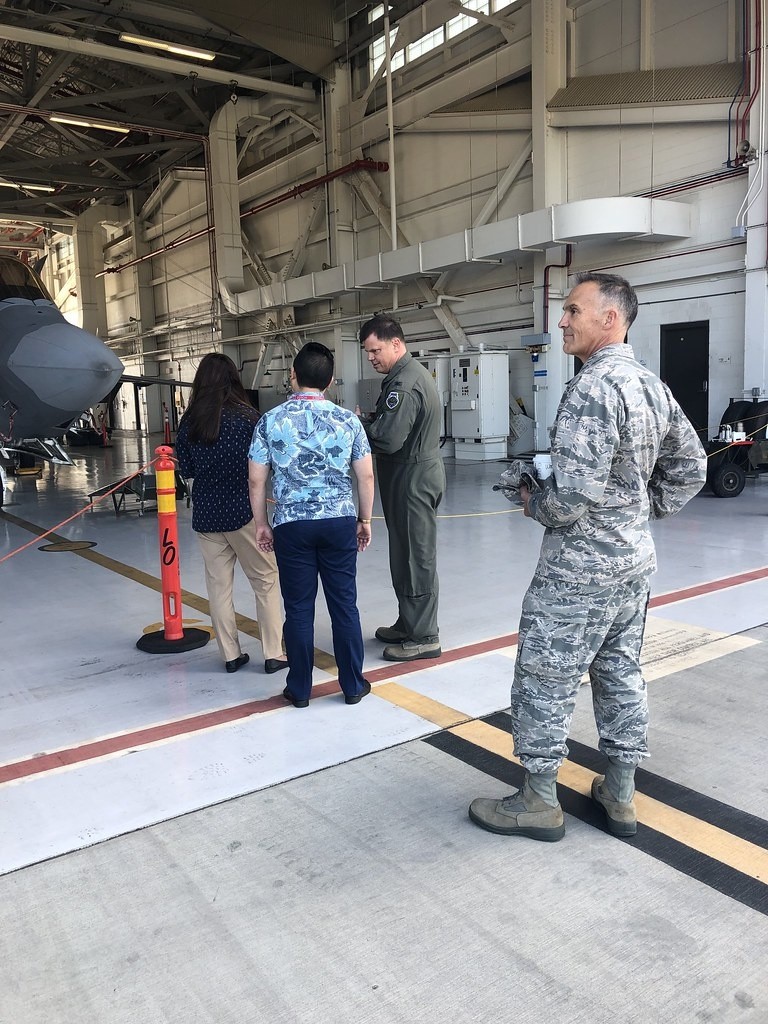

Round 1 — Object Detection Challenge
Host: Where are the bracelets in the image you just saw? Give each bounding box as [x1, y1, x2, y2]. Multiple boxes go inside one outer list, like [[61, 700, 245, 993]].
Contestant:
[[358, 516, 371, 524]]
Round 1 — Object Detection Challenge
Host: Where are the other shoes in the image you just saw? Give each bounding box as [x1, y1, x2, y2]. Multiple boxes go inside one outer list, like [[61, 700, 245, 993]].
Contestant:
[[345, 679, 371, 704], [284, 686, 309, 708], [226, 653, 249, 673], [265, 654, 290, 674]]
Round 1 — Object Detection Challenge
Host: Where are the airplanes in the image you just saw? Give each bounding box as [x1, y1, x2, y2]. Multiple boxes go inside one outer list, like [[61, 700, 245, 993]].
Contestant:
[[1, 253, 194, 476]]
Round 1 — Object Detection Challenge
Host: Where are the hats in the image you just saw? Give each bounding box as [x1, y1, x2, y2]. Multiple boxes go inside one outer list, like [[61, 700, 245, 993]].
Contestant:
[[497, 459, 545, 507]]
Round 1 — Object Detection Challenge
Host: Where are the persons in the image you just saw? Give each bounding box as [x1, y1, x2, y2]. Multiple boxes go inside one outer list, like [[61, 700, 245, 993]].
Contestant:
[[354, 315, 448, 663], [247, 342, 375, 708], [470, 274, 708, 842], [175, 352, 289, 674]]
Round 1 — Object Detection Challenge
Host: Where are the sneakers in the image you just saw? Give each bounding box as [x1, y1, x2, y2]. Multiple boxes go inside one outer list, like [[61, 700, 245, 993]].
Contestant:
[[375, 626, 439, 643], [383, 640, 441, 661]]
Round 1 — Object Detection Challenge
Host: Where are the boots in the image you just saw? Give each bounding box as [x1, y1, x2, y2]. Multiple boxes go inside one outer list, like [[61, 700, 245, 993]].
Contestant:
[[469, 768, 566, 842], [591, 756, 637, 837]]
